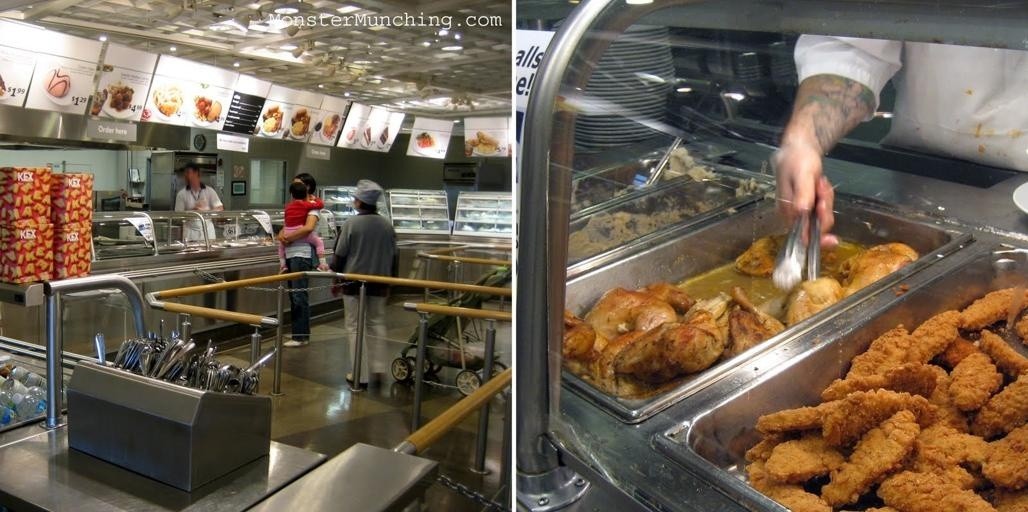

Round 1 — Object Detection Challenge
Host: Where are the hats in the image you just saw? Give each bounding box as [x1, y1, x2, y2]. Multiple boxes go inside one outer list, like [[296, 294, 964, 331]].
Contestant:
[[348, 179, 384, 205]]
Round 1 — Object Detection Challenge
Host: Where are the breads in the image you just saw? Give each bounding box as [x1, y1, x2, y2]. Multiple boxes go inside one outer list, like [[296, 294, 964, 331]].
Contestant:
[[44, 68, 71, 98]]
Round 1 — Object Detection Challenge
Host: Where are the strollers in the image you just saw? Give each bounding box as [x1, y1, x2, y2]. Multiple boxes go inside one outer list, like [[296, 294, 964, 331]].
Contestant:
[[387, 263, 512, 400]]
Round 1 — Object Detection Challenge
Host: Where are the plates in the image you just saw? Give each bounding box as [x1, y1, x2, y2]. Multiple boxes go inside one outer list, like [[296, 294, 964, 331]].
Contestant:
[[42, 67, 77, 106], [102, 82, 138, 120], [473, 143, 502, 157], [0, 57, 18, 101], [189, 94, 223, 127], [346, 125, 389, 149], [150, 85, 184, 122], [414, 132, 443, 155], [260, 108, 341, 143], [552, 15, 677, 150]]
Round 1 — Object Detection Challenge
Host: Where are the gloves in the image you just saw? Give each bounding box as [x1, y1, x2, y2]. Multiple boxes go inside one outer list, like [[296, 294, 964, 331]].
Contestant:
[[330, 279, 341, 298]]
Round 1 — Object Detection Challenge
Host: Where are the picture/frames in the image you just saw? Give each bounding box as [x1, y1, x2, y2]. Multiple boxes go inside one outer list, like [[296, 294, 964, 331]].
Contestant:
[[231, 181, 246, 196]]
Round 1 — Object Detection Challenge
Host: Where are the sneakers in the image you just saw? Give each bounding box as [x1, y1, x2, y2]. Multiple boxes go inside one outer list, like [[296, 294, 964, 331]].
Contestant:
[[317, 264, 333, 272], [279, 264, 288, 274], [282, 339, 310, 347], [347, 372, 368, 391]]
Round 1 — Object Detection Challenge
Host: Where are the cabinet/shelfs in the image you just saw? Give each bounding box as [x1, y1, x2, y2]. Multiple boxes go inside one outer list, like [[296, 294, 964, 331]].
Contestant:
[[314, 183, 512, 244]]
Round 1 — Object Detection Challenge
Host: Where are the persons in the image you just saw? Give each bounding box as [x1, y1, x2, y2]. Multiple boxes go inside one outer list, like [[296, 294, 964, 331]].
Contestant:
[[770, 30, 1028, 242], [278, 173, 318, 348], [331, 179, 399, 388], [173, 163, 223, 246], [277, 181, 331, 275]]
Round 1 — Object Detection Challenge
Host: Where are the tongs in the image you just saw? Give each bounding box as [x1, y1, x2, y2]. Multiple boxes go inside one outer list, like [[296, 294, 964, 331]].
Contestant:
[[771, 206, 820, 292]]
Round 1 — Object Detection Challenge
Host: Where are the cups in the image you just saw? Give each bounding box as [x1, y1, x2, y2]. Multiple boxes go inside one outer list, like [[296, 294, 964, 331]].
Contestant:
[[92, 91, 107, 115]]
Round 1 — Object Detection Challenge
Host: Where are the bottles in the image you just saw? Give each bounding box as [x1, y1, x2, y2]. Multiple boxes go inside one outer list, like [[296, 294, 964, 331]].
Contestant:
[[0, 353, 67, 425]]
